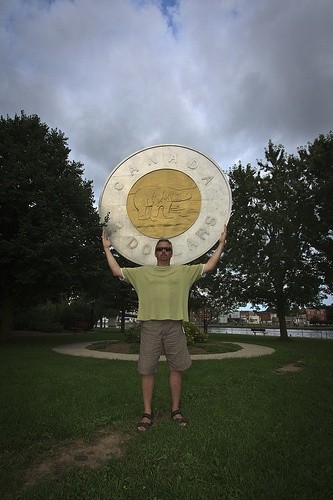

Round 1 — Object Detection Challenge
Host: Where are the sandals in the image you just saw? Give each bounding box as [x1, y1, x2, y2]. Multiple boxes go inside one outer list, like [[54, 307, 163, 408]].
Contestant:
[[136, 413, 154, 432], [170, 410, 188, 427]]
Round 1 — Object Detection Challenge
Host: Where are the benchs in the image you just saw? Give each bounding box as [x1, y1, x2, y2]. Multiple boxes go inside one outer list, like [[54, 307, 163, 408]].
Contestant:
[[251, 328, 268, 336], [73, 322, 93, 336]]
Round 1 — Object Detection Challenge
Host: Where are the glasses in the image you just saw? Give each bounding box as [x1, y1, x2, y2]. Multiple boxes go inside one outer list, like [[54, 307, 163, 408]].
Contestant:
[[155, 247, 172, 251]]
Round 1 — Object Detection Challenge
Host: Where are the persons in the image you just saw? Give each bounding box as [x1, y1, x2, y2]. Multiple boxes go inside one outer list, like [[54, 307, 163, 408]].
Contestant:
[[101, 224, 228, 433]]
